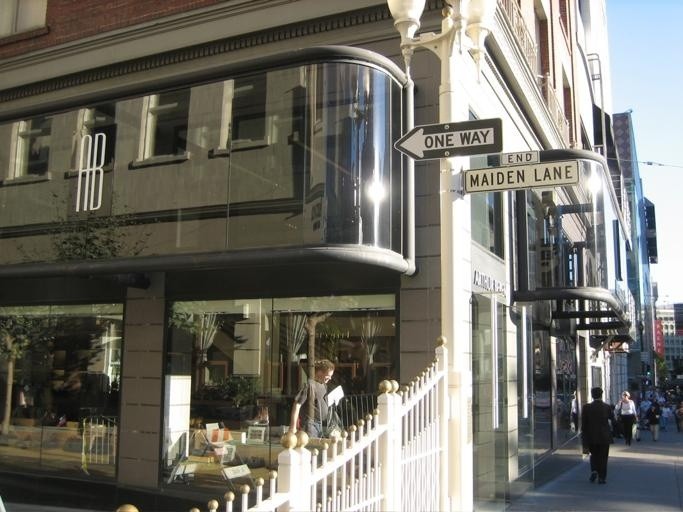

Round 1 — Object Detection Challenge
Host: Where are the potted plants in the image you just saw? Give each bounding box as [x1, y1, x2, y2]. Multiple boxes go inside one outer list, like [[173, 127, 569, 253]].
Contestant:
[[210, 371, 258, 431]]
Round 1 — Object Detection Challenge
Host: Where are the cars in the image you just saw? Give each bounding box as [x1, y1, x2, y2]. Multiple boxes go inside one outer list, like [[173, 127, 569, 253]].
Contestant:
[[665, 388, 682, 407]]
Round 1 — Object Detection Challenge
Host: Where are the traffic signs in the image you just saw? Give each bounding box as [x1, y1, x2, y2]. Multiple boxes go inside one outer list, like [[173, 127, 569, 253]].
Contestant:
[[393, 117, 502, 162]]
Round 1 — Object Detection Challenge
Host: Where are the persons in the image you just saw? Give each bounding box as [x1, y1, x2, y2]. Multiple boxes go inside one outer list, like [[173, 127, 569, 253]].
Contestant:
[[581, 387, 617, 485], [643, 394, 683, 441], [615, 391, 638, 446], [570, 392, 579, 433], [286, 358, 344, 439]]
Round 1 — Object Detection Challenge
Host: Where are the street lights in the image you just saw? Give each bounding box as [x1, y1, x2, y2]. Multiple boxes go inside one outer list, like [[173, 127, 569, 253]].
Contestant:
[[637, 320, 645, 401]]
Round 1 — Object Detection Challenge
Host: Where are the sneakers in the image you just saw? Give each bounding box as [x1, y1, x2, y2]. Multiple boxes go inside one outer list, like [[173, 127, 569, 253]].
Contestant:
[[624, 439, 659, 446], [589, 471, 597, 484], [598, 474, 607, 485]]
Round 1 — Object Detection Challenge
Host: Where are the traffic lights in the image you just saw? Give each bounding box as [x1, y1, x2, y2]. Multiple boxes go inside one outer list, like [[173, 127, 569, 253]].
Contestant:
[[646, 365, 650, 375]]
